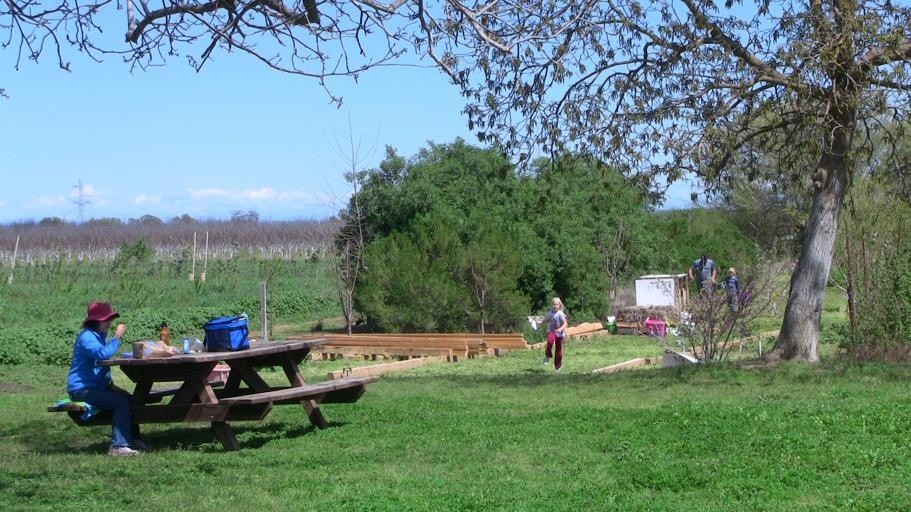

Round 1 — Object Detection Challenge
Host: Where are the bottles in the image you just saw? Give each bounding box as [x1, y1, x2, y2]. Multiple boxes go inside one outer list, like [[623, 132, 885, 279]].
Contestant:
[[159, 320, 170, 346]]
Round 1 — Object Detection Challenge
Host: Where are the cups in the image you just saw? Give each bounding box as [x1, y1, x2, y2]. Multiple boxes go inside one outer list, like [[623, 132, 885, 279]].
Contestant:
[[132, 343, 144, 359], [182, 338, 190, 354]]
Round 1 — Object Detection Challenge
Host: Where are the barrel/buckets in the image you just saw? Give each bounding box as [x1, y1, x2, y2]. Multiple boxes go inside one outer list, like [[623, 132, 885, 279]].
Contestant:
[[604, 322, 617, 334]]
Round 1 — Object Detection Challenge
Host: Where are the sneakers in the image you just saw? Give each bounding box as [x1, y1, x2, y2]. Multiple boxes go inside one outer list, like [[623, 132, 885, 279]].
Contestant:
[[107, 446, 137, 456], [554, 368, 560, 373], [133, 438, 144, 449], [542, 356, 548, 365]]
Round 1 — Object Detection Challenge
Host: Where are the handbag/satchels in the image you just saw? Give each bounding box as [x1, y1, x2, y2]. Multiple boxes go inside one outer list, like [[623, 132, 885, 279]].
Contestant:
[[199, 314, 249, 352], [133, 341, 183, 359]]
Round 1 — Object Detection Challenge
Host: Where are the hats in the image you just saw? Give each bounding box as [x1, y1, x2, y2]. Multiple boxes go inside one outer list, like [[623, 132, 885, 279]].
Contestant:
[[82, 302, 119, 326]]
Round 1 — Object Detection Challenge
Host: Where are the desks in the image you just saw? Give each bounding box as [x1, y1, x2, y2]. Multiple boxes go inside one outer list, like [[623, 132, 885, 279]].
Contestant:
[[99, 337, 332, 450]]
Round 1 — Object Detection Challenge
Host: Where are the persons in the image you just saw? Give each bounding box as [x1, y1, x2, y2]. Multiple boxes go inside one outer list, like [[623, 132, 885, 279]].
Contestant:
[[67, 301, 150, 456], [688, 253, 717, 309], [537, 296, 568, 374], [713, 267, 740, 314]]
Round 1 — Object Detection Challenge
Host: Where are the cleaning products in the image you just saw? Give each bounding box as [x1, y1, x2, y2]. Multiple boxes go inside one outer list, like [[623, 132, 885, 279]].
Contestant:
[[159, 321, 170, 343]]
